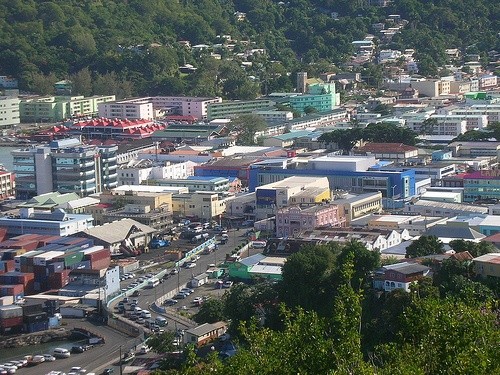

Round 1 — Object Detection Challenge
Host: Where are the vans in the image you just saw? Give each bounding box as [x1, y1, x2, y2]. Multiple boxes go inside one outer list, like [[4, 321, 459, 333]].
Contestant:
[[72, 346, 84, 353], [54, 347, 70, 358], [42, 353, 56, 361]]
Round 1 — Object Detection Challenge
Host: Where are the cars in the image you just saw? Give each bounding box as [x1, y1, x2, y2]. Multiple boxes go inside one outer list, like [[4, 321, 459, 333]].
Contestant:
[[115, 216, 270, 369], [48, 370, 62, 375], [103, 368, 114, 375], [0, 355, 44, 375]]
